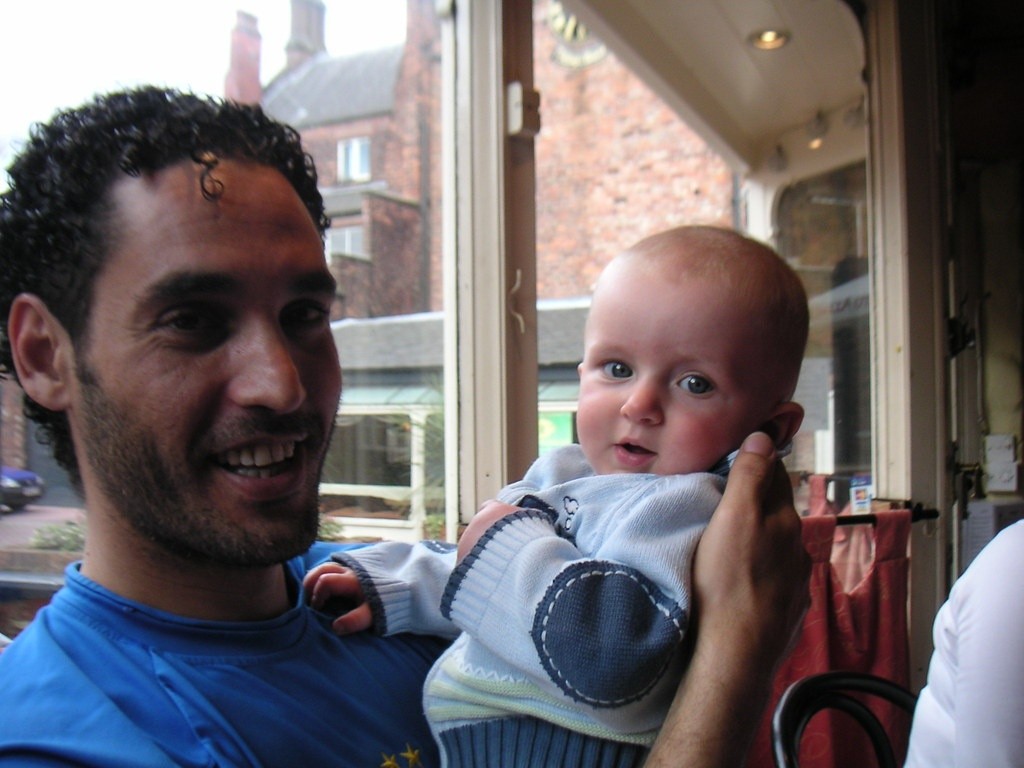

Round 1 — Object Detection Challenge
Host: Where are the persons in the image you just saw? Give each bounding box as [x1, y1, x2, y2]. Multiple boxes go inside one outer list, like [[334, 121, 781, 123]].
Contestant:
[[304, 227, 809, 768], [0, 88, 808, 768], [903, 514, 1024, 768]]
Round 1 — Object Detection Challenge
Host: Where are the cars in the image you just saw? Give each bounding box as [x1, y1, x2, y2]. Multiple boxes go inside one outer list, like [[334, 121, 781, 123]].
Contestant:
[[0, 460, 47, 511]]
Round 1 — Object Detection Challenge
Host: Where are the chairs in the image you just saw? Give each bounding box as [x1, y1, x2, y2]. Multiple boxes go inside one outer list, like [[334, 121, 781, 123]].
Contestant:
[[773, 669, 918, 768]]
